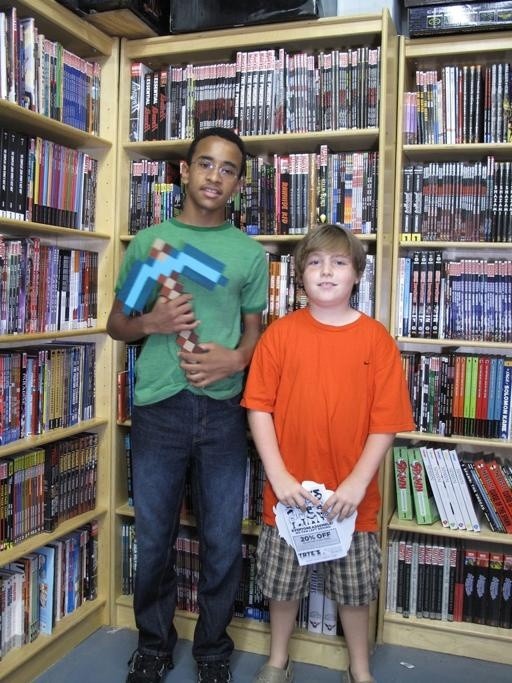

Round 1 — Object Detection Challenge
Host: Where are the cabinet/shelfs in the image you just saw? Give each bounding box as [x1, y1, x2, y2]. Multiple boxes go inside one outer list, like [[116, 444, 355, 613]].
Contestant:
[[377, 26, 511, 666], [114, 6, 400, 671], [0, 1, 118, 683]]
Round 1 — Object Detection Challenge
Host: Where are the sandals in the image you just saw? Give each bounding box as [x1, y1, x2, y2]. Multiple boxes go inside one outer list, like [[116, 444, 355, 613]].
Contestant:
[[251, 653, 295, 683], [342, 664, 378, 683]]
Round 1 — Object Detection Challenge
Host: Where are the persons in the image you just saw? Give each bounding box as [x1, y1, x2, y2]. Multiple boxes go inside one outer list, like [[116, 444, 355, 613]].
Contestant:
[[105, 126, 272, 683], [238, 223, 418, 683]]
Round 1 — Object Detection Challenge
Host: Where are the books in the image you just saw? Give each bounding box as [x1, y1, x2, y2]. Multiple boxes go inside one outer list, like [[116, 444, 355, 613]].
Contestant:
[[272, 144, 377, 235], [242, 543, 271, 622], [272, 250, 309, 320], [128, 157, 186, 236], [123, 433, 135, 507], [387, 531, 417, 620], [403, 155, 511, 242], [173, 531, 202, 614], [1, 431, 100, 554], [119, 522, 137, 593], [408, 440, 512, 533], [0, 231, 100, 336], [395, 250, 416, 338], [407, 1, 512, 39], [0, 520, 100, 661], [292, 572, 344, 638], [1, 125, 99, 232], [418, 535, 512, 630], [115, 343, 138, 425], [349, 253, 375, 321], [0, 5, 103, 137], [394, 446, 413, 520], [418, 248, 511, 345], [128, 44, 382, 141], [403, 62, 512, 144], [222, 152, 270, 236], [404, 0, 482, 8], [399, 349, 417, 430], [418, 351, 512, 443], [260, 249, 271, 332], [1, 340, 97, 446], [242, 453, 268, 527]]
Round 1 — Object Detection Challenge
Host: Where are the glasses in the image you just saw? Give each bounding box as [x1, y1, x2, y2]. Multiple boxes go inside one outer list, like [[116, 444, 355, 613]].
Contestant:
[[189, 158, 237, 183]]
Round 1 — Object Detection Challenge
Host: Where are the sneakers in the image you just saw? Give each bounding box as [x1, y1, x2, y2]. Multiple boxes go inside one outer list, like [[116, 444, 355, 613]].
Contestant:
[[125, 649, 174, 683], [196, 659, 232, 683]]
